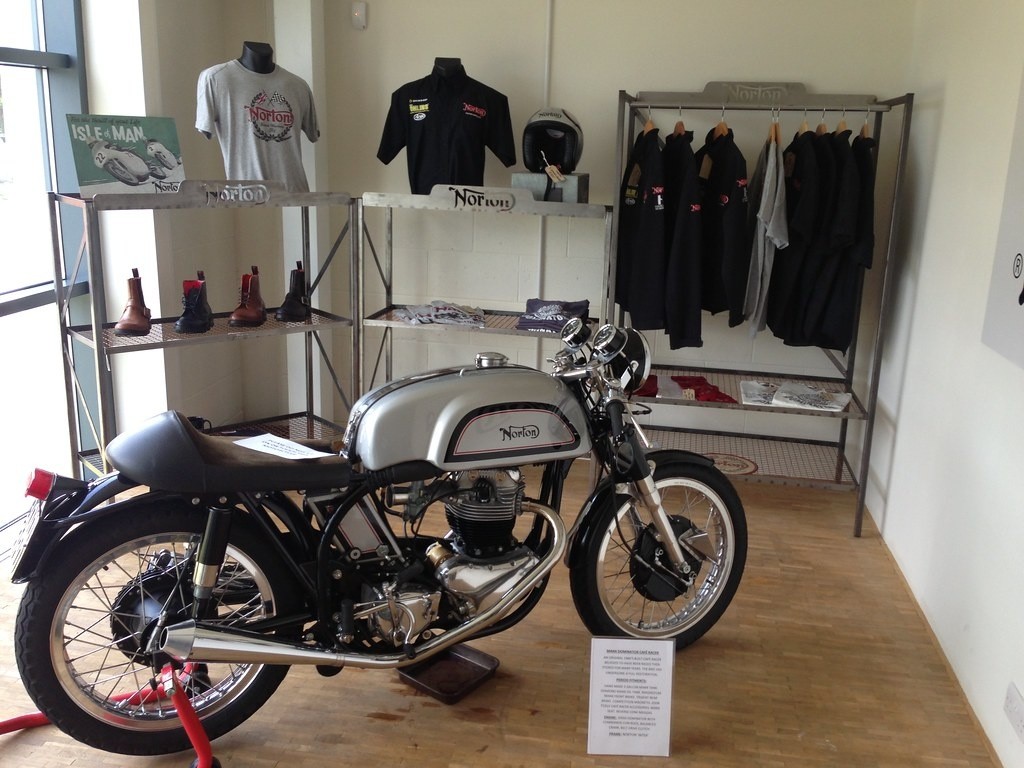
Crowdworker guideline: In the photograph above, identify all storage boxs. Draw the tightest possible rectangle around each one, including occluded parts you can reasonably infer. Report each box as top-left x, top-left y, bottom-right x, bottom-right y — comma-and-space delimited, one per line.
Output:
512, 172, 589, 204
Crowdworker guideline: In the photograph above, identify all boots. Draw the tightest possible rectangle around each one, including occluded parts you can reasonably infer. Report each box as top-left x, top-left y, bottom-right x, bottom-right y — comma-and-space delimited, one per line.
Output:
114, 268, 151, 336
174, 271, 214, 334
228, 266, 267, 327
276, 261, 311, 322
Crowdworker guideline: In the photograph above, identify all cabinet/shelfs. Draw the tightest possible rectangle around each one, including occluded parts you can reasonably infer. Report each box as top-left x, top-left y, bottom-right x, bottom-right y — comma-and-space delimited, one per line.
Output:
591, 79, 914, 538
48, 179, 359, 506
358, 193, 614, 461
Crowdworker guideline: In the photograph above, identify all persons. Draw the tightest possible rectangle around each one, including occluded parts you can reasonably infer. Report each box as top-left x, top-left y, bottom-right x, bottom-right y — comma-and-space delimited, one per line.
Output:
142, 136, 159, 150
85, 135, 123, 151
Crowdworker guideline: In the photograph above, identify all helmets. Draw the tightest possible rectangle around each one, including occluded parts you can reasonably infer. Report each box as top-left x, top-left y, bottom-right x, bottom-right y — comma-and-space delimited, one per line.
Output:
522, 107, 584, 175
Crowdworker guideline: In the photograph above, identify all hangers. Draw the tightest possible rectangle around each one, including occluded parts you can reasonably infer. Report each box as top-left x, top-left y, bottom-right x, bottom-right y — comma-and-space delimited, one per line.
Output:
637, 103, 877, 138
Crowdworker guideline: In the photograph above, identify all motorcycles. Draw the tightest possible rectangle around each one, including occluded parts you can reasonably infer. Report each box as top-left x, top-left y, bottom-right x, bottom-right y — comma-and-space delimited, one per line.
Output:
9, 301, 749, 757
